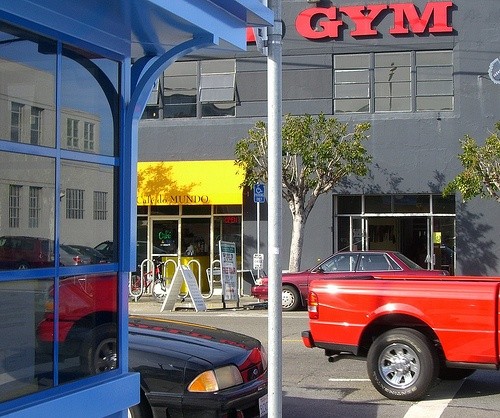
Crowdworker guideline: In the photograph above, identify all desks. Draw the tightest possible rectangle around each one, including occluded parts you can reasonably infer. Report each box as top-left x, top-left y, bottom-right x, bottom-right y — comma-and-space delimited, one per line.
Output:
161, 254, 210, 295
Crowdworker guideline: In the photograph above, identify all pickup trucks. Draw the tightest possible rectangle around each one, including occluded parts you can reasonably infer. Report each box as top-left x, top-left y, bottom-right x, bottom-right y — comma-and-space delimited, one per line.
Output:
36, 273, 118, 377
301, 275, 500, 402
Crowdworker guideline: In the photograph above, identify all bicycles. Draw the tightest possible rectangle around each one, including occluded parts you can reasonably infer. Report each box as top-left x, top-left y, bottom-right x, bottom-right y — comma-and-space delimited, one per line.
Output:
128, 257, 168, 303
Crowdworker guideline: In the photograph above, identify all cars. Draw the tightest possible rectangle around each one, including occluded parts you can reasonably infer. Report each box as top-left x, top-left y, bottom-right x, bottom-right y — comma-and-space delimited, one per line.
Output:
127, 314, 268, 418
251, 250, 451, 311
0, 234, 171, 279
